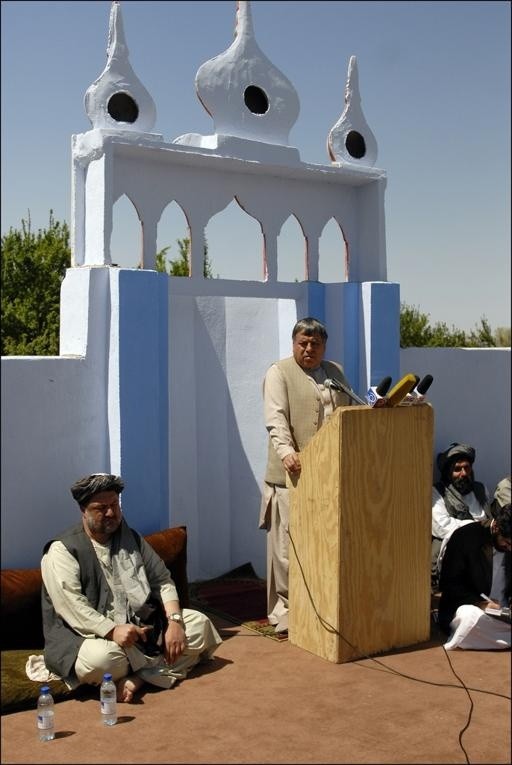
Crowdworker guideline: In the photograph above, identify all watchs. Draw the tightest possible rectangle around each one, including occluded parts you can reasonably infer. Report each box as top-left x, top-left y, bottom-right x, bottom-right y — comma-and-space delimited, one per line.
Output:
165, 613, 184, 624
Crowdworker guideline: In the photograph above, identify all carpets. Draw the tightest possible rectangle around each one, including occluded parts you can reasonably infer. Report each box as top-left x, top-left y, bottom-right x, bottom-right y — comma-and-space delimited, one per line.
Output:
188, 562, 290, 642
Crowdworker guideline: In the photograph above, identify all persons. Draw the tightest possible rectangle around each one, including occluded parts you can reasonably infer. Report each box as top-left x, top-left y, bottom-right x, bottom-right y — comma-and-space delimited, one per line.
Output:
40, 473, 223, 703
257, 310, 355, 639
431, 441, 512, 636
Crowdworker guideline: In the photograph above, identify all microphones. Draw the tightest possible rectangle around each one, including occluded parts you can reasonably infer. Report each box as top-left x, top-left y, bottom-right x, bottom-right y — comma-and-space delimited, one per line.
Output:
366, 375, 393, 407
324, 379, 344, 393
399, 374, 421, 407
412, 374, 434, 405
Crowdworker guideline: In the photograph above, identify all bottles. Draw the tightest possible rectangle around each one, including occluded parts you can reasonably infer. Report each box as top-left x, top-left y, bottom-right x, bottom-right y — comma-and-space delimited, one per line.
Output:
100, 673, 117, 725
38, 686, 56, 742
